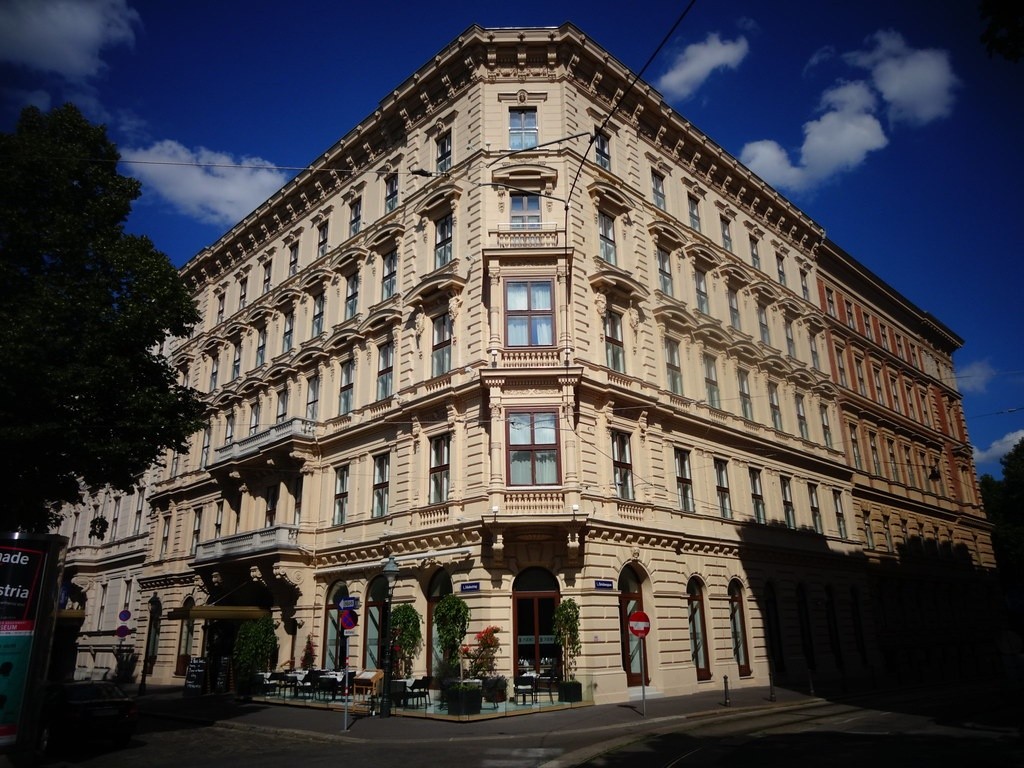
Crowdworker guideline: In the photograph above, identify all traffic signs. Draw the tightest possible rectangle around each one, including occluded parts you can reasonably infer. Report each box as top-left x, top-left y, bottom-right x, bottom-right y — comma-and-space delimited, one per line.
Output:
335, 595, 360, 614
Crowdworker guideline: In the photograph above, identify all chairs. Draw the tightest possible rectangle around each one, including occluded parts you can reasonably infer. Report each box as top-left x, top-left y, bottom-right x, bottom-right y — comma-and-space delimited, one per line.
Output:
249, 673, 555, 709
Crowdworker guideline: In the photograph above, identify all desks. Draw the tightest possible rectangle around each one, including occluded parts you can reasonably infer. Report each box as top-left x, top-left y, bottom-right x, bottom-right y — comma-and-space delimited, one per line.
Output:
258, 673, 277, 684
320, 674, 344, 693
463, 678, 483, 690
393, 679, 434, 705
285, 674, 313, 697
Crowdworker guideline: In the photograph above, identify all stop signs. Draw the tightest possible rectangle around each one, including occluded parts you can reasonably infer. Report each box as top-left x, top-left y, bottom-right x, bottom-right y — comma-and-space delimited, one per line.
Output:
628, 611, 651, 638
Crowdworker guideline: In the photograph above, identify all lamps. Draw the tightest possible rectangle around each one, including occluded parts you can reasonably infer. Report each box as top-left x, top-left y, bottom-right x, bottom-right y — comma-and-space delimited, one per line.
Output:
572, 504, 579, 514
492, 506, 499, 516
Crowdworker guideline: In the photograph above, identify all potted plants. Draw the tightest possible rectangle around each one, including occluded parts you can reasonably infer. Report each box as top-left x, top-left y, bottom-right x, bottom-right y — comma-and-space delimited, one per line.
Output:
551, 596, 583, 702
431, 592, 483, 715
232, 614, 280, 695
389, 601, 426, 708
